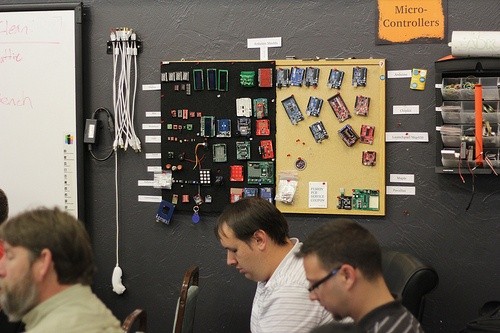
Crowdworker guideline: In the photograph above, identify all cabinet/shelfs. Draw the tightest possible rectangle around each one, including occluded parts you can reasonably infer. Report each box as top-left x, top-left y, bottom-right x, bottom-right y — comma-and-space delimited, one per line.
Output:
435, 56, 500, 174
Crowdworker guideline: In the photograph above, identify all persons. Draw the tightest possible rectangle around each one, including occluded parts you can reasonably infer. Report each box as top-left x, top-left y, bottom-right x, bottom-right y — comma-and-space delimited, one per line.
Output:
214, 195, 354, 333
0, 207, 129, 333
0, 187, 10, 269
294, 217, 425, 333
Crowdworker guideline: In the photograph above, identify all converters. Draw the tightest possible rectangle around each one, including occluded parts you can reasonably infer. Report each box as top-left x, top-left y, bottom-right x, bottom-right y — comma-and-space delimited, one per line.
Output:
84, 118, 98, 144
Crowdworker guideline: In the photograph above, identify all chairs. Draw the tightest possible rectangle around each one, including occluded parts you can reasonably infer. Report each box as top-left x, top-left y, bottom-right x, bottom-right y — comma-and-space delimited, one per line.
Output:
121, 267, 201, 333
381, 250, 438, 321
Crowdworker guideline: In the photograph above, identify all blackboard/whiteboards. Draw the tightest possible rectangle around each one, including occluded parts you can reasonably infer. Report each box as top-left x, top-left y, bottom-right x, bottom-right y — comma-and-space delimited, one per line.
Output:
0, 2, 83, 225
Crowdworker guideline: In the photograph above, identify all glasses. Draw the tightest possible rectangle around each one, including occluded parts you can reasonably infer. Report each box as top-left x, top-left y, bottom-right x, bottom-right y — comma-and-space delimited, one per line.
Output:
308, 265, 341, 293
